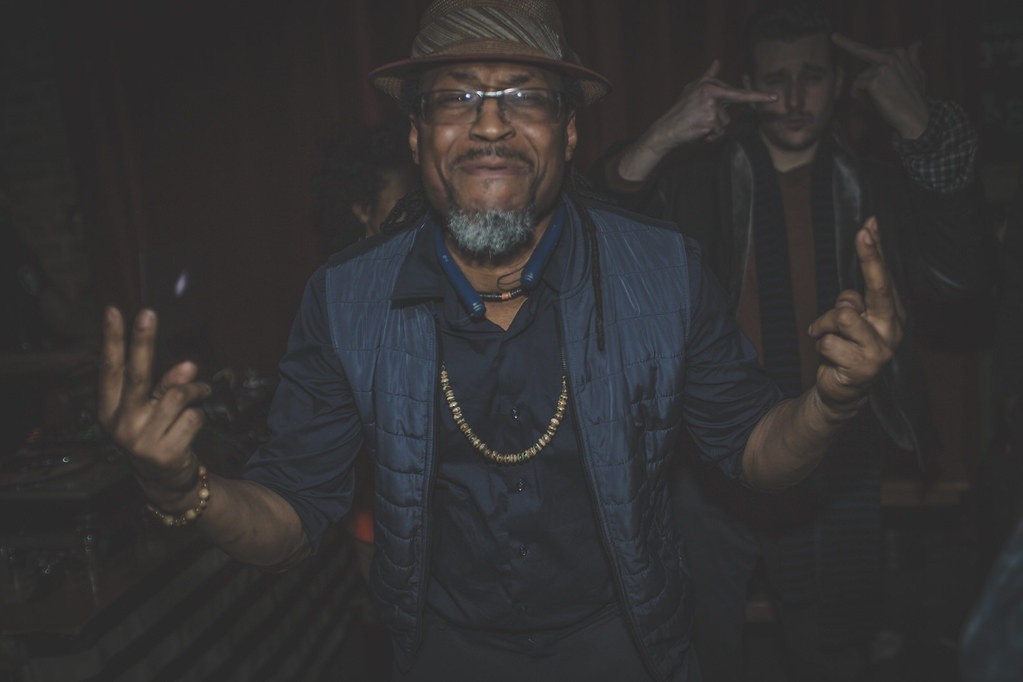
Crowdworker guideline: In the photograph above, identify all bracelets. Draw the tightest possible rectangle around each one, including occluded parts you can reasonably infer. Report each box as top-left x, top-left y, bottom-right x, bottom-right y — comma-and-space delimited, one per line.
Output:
146, 461, 210, 528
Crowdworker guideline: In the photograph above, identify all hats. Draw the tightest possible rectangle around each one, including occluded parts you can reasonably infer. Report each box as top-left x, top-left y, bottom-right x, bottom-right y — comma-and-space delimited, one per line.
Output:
370, 0, 613, 109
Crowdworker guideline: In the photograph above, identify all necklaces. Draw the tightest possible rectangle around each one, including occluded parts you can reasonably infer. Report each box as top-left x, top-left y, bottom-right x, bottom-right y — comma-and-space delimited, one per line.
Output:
478, 285, 527, 302
437, 356, 570, 465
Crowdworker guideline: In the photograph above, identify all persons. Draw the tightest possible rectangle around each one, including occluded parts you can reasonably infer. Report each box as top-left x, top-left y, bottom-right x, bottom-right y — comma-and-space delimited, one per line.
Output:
334, 119, 416, 584
96, 0, 907, 681
596, 0, 978, 681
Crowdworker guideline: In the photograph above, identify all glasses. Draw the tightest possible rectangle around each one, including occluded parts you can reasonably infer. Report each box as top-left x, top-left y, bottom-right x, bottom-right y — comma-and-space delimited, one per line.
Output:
410, 88, 571, 125
